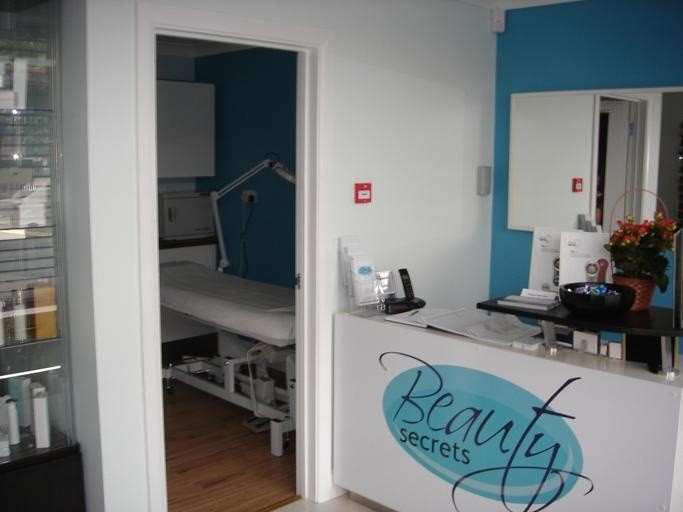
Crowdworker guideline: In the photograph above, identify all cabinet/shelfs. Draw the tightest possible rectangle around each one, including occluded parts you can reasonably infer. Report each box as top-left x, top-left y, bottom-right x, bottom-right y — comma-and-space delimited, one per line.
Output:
0, 3, 87, 511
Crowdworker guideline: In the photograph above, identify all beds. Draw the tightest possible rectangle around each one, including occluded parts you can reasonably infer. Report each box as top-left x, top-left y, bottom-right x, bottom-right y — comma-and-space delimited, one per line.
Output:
160, 260, 294, 457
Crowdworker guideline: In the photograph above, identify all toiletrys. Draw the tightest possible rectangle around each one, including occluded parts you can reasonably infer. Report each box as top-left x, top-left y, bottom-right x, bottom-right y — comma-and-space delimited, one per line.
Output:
0, 281, 56, 460
0, 55, 12, 91
11, 58, 28, 110
0, 89, 16, 110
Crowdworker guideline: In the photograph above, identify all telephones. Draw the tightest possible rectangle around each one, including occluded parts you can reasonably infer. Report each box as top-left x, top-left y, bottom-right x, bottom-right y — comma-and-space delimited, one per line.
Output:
384, 268, 426, 314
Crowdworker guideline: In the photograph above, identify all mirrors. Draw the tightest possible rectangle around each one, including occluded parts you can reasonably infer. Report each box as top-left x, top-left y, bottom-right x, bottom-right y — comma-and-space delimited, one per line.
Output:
593, 91, 682, 232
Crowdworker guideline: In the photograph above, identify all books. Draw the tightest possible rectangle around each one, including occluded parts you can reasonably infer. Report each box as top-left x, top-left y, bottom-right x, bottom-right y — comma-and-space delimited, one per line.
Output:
418, 306, 542, 347
558, 230, 616, 288
497, 295, 559, 312
527, 223, 584, 295
384, 307, 455, 329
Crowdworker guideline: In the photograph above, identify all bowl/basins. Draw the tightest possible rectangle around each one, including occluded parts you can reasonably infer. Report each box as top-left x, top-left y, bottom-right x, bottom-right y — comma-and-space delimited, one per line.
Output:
559, 280, 637, 316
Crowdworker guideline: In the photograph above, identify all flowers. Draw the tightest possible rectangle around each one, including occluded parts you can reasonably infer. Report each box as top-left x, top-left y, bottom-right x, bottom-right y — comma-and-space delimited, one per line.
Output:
602, 213, 675, 293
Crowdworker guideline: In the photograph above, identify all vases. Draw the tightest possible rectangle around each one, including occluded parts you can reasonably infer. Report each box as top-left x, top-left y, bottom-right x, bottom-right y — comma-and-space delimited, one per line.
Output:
610, 274, 653, 310
